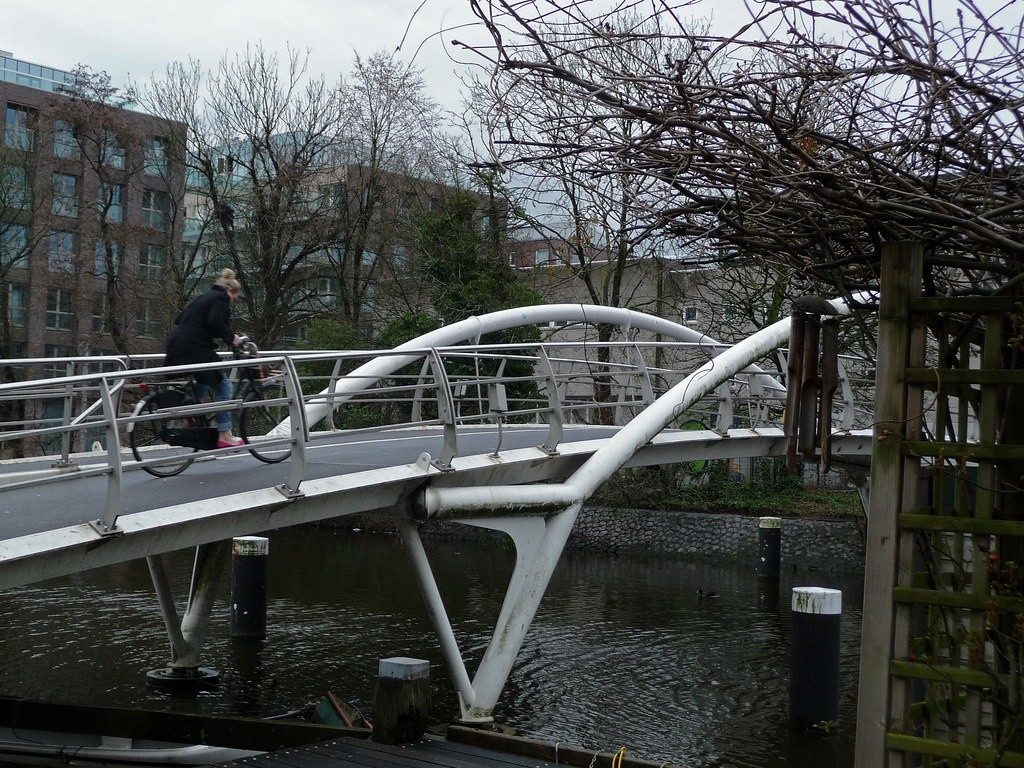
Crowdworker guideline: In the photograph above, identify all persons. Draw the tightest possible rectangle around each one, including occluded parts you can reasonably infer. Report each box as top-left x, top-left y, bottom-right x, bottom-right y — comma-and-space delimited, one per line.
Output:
166, 267, 244, 448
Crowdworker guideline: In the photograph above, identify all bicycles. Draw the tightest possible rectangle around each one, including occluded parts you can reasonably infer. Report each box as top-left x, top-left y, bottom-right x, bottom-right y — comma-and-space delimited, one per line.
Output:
126, 334, 308, 479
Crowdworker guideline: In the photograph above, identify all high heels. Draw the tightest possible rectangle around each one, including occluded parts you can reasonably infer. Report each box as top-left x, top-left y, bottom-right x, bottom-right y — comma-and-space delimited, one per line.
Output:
217, 440, 244, 456
